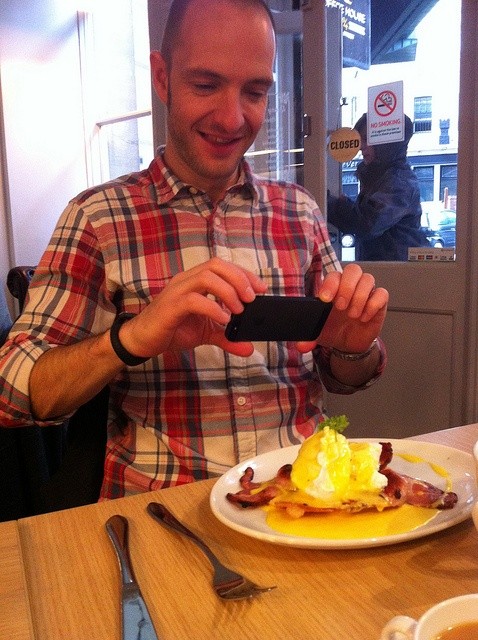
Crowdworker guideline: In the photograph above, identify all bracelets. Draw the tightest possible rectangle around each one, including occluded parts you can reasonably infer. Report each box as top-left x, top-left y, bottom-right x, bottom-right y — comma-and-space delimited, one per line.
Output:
110, 312, 147, 367
327, 340, 381, 363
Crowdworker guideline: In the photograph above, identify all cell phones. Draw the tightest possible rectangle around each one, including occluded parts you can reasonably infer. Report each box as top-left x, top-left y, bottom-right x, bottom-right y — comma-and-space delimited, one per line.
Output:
224, 295, 332, 343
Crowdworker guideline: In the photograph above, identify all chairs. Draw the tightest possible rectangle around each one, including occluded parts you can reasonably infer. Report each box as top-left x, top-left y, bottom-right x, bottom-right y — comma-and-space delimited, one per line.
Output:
6, 266, 107, 512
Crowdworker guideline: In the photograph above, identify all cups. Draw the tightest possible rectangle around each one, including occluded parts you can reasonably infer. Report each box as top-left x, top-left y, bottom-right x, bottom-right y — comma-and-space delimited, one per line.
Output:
381, 592, 477, 640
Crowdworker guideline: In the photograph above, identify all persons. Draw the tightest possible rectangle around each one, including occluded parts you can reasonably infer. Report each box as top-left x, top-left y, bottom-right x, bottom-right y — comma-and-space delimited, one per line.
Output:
0, 0, 390, 506
326, 111, 431, 263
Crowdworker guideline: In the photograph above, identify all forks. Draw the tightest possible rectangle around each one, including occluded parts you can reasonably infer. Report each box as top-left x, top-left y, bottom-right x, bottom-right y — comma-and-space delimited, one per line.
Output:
145, 501, 279, 602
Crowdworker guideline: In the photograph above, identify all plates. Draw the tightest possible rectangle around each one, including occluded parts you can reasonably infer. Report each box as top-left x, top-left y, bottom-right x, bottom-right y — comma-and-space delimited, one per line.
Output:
209, 437, 478, 553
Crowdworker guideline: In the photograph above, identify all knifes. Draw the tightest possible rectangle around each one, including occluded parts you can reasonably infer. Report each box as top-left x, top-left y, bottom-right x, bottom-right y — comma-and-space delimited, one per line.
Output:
104, 514, 161, 639
